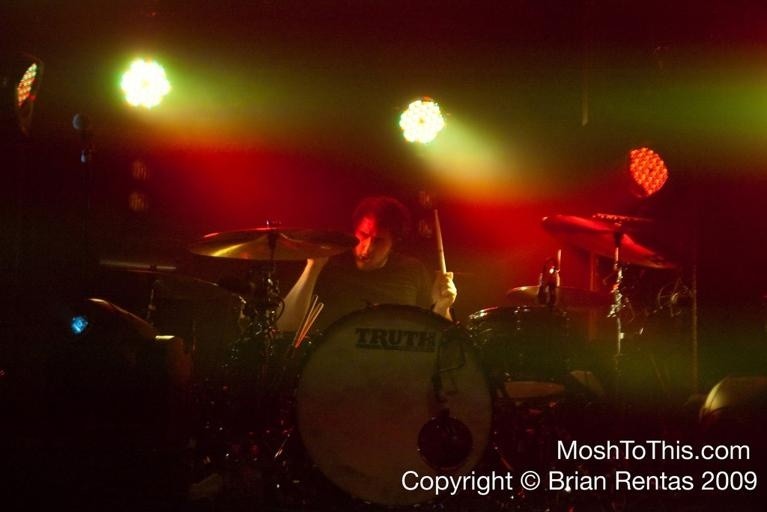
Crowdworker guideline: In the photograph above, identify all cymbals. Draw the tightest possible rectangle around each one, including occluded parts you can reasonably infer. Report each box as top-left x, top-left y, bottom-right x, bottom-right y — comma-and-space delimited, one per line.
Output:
505, 285, 606, 308
542, 212, 674, 270
90, 269, 228, 301
184, 227, 357, 262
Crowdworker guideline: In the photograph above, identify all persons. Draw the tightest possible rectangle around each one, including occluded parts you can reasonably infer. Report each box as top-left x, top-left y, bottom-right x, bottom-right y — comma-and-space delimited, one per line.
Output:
274, 195, 457, 338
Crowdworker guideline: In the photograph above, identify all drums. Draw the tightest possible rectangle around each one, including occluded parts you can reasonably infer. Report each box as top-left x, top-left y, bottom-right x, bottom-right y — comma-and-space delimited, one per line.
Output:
462, 304, 570, 399
278, 304, 492, 509
